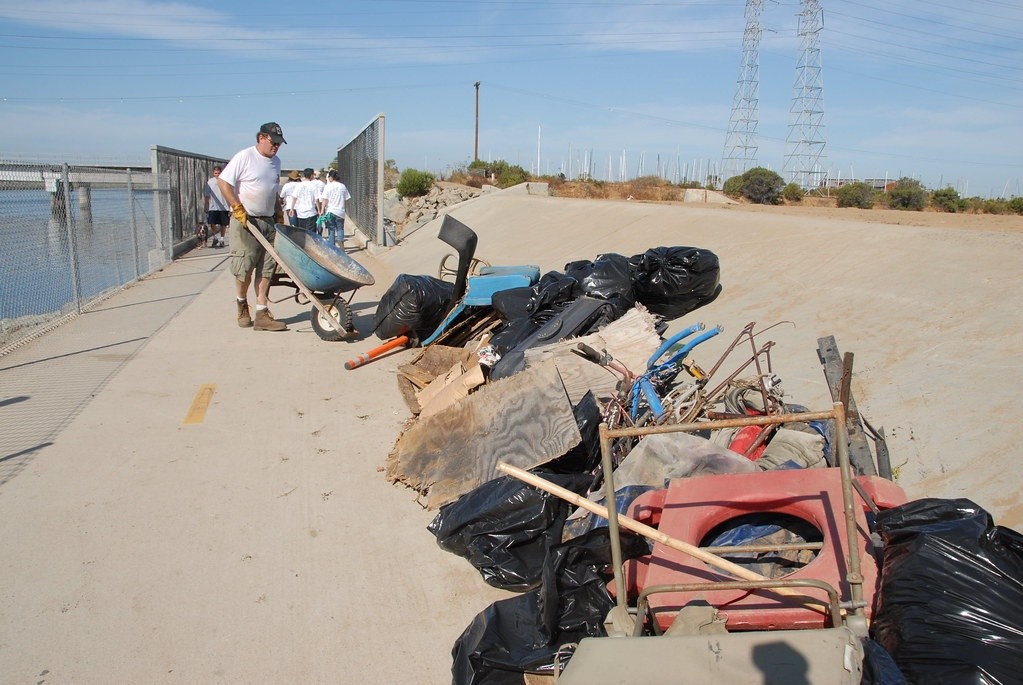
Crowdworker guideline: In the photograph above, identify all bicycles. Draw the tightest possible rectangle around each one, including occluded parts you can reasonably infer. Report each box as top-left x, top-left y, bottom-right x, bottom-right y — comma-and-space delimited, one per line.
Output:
561, 321, 783, 492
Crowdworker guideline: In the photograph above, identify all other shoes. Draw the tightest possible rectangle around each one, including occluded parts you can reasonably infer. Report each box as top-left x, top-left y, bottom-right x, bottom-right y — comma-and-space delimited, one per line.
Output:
220, 241, 224, 247
212, 238, 218, 247
339, 242, 346, 252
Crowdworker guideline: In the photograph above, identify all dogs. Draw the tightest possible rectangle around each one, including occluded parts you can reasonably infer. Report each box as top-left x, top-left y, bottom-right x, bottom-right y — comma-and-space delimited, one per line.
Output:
196, 223, 208, 249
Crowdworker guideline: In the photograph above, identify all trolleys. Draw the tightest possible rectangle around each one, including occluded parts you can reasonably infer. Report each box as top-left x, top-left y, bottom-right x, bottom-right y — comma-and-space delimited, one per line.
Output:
244, 219, 376, 343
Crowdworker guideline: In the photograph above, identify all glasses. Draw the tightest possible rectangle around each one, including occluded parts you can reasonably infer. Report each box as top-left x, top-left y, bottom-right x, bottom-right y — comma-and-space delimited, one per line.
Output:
262, 133, 281, 146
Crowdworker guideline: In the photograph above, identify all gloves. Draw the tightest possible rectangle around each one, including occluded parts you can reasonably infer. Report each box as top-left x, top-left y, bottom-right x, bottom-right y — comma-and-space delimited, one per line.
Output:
276, 210, 285, 224
232, 203, 249, 229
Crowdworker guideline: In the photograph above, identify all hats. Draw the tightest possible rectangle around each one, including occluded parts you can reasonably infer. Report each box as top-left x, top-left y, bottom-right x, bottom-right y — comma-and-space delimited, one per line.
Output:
261, 122, 287, 144
288, 171, 301, 180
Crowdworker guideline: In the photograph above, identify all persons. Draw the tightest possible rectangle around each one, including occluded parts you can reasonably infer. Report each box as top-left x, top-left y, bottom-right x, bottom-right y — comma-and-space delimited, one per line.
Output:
279, 168, 351, 253
217, 121, 287, 330
204, 166, 231, 248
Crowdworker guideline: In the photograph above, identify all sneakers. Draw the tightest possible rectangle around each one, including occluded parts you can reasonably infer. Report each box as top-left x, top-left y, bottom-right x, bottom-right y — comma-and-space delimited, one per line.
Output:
254, 308, 287, 331
237, 299, 252, 328
